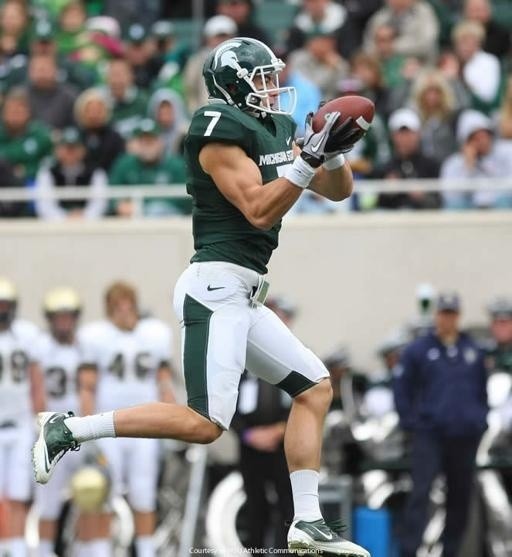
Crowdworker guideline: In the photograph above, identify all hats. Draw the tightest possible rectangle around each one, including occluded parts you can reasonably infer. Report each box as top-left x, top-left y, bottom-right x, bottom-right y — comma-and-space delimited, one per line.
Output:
456, 111, 492, 144
205, 16, 236, 38
437, 293, 459, 315
387, 108, 421, 134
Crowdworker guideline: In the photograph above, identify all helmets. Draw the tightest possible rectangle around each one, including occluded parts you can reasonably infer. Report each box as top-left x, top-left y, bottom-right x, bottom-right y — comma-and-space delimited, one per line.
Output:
0, 278, 16, 306
202, 37, 298, 116
39, 285, 80, 317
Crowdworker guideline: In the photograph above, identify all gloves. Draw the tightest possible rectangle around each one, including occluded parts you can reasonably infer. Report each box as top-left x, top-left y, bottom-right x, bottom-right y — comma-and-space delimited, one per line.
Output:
295, 98, 365, 169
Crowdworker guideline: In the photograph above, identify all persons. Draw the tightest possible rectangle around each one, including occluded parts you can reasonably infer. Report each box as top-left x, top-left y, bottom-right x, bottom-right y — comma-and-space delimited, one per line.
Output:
0, 0, 512, 557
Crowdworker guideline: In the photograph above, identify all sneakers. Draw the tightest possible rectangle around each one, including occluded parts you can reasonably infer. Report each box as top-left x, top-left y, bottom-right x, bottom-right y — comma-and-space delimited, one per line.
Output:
32, 411, 81, 484
287, 517, 371, 557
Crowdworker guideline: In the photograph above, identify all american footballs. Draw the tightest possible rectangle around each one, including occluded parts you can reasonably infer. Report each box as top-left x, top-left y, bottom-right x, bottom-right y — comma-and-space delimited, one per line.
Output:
308, 96, 374, 152
